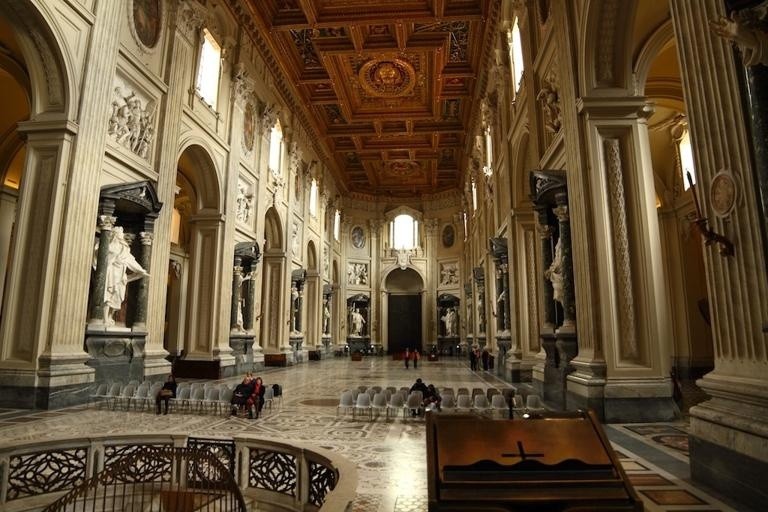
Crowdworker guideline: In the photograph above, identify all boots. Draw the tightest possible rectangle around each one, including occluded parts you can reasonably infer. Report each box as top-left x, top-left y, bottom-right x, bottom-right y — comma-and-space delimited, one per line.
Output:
253, 412, 259, 419
247, 410, 253, 419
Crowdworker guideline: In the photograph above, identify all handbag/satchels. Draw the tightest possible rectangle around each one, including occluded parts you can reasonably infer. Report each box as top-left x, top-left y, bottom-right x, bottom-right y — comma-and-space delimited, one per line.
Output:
160, 388, 171, 396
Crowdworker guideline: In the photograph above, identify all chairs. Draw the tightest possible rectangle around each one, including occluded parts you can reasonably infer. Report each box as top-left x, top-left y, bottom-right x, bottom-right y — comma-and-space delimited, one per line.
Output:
337, 385, 545, 422
89, 379, 286, 419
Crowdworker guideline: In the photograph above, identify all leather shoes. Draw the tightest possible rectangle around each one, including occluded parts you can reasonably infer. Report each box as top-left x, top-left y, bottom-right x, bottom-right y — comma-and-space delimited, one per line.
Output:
233, 407, 237, 413
230, 412, 237, 416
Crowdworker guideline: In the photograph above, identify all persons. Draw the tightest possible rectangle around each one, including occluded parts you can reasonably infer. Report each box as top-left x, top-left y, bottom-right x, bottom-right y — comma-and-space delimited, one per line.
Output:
409, 378, 442, 416
229, 371, 265, 419
546, 233, 564, 306
441, 308, 457, 336
102, 226, 147, 327
412, 348, 420, 368
469, 348, 489, 372
156, 375, 176, 415
404, 348, 410, 368
352, 307, 366, 336
